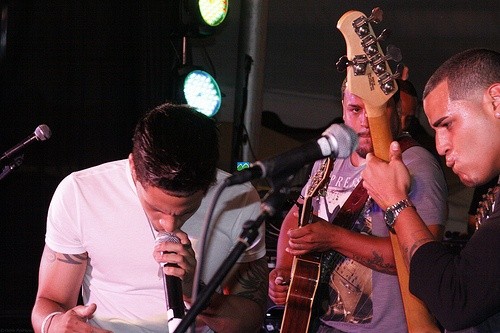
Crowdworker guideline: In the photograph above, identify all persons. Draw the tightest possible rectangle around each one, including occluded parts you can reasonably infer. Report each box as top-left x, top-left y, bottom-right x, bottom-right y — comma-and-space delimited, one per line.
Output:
268, 58, 448, 333
30, 104, 269, 333
359, 47, 500, 332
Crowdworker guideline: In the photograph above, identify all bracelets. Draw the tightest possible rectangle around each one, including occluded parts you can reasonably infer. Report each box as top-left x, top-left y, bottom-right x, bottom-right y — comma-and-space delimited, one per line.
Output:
41, 312, 63, 333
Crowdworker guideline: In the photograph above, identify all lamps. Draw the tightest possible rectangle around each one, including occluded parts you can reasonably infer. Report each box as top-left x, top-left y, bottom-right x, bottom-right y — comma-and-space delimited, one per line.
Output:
168, 0, 233, 123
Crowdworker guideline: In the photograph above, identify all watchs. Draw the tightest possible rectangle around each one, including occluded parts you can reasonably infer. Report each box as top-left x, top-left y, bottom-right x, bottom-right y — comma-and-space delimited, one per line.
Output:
384, 200, 416, 234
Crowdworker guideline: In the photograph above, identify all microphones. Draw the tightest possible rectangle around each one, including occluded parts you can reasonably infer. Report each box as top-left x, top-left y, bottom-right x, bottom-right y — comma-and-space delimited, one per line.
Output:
0, 124, 52, 167
224, 123, 358, 187
155, 230, 186, 333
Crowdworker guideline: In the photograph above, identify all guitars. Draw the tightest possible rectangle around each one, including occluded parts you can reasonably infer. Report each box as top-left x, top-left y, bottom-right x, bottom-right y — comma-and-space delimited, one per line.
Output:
336, 7, 446, 333
280, 158, 334, 333
